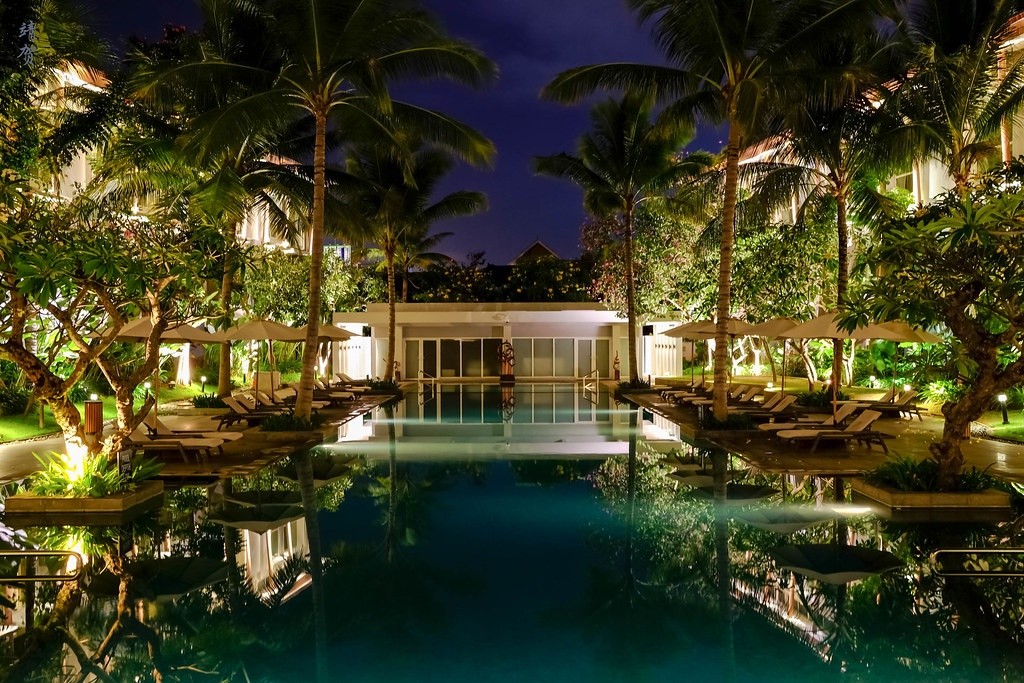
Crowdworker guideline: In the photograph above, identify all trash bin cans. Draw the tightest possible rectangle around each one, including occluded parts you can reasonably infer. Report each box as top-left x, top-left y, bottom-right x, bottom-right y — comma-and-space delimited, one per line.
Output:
84, 400, 103, 435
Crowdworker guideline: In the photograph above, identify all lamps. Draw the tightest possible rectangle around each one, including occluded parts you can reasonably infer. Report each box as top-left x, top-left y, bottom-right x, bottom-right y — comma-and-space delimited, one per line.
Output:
201, 376, 208, 382
870, 376, 875, 382
998, 393, 1007, 403
903, 384, 911, 392
144, 382, 151, 389
90, 393, 99, 400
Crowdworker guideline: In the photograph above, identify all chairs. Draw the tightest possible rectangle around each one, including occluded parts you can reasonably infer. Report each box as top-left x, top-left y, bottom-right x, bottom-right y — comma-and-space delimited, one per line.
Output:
110, 373, 372, 465
650, 376, 928, 456
